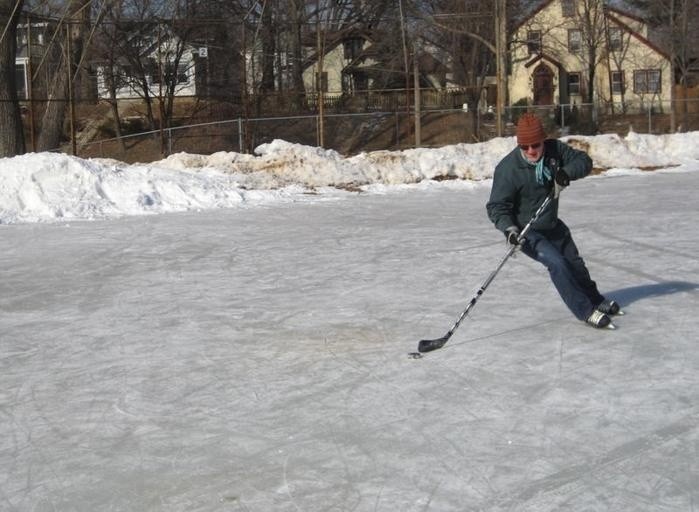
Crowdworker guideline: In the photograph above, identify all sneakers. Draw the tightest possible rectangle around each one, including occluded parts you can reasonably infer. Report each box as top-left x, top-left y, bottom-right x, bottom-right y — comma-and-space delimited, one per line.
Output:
598, 298, 619, 315
585, 308, 610, 328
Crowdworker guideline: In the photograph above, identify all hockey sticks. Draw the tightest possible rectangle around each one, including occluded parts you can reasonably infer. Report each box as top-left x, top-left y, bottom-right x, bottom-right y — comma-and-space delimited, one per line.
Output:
419, 185, 556, 353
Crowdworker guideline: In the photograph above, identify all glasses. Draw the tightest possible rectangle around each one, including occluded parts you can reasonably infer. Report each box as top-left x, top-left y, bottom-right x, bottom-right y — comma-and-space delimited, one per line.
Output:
518, 143, 540, 150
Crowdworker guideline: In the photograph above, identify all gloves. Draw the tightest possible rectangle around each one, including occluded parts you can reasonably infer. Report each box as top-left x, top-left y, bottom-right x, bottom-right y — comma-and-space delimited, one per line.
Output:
504, 225, 526, 258
555, 168, 569, 190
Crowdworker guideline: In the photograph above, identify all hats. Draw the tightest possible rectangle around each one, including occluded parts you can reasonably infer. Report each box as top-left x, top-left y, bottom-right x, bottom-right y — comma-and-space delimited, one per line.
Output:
517, 113, 544, 144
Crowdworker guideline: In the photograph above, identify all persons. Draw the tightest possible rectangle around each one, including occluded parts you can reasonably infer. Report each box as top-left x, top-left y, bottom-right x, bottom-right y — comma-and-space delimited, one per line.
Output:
483, 112, 621, 328
